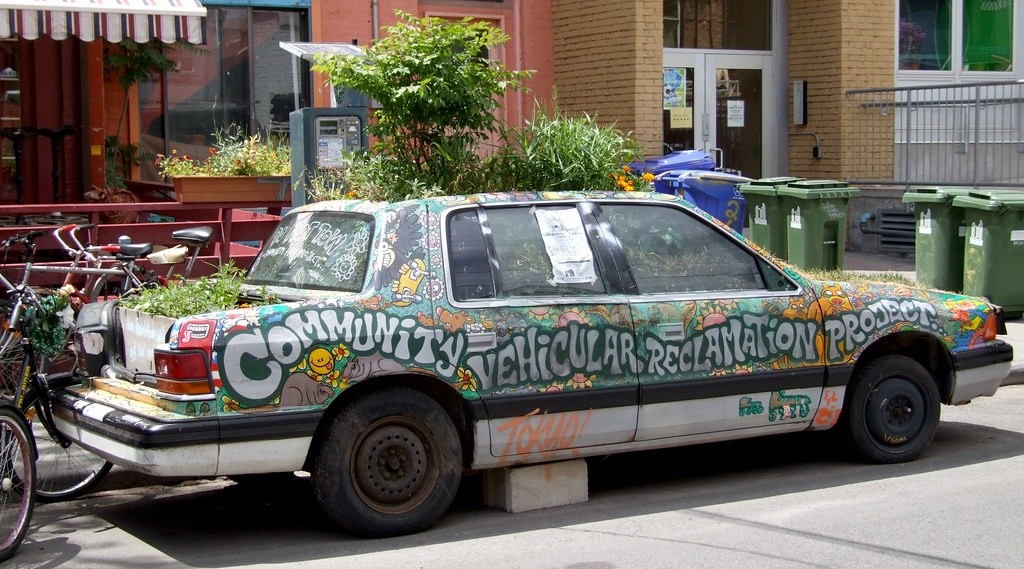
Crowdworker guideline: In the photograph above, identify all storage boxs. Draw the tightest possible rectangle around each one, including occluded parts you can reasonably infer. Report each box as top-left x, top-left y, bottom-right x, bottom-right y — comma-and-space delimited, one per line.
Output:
475, 459, 589, 512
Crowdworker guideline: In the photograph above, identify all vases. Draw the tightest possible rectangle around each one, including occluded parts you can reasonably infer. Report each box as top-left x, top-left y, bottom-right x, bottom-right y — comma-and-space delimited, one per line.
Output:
171, 173, 291, 203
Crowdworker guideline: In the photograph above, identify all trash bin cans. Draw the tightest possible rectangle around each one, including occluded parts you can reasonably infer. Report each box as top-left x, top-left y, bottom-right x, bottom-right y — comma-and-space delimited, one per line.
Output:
952, 188, 1024, 320
902, 186, 976, 294
655, 170, 754, 236
629, 150, 716, 176
777, 180, 861, 275
735, 177, 807, 265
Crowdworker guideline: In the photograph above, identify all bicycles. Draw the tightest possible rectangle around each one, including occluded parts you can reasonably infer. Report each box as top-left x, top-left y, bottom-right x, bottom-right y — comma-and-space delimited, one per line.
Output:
0, 224, 214, 560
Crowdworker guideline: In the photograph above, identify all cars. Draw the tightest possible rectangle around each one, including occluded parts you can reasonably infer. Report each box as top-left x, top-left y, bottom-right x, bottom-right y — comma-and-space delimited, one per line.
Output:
46, 191, 1013, 538
147, 25, 309, 147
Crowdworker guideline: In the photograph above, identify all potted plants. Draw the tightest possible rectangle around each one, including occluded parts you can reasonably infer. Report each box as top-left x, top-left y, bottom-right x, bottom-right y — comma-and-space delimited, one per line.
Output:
118, 256, 280, 376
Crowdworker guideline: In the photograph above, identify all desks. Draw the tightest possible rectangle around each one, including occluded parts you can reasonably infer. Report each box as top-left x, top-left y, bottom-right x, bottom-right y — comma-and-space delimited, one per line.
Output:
120, 179, 176, 202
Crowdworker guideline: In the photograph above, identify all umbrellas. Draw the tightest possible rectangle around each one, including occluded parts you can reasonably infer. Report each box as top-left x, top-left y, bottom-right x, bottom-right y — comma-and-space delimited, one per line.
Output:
148, 22, 305, 138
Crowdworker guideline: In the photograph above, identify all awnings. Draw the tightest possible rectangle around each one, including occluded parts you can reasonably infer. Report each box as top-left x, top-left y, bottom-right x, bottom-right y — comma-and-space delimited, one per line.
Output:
0, 0, 208, 45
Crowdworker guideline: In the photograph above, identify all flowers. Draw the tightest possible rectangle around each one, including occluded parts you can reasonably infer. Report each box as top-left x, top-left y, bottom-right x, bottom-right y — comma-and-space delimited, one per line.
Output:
153, 137, 282, 180
